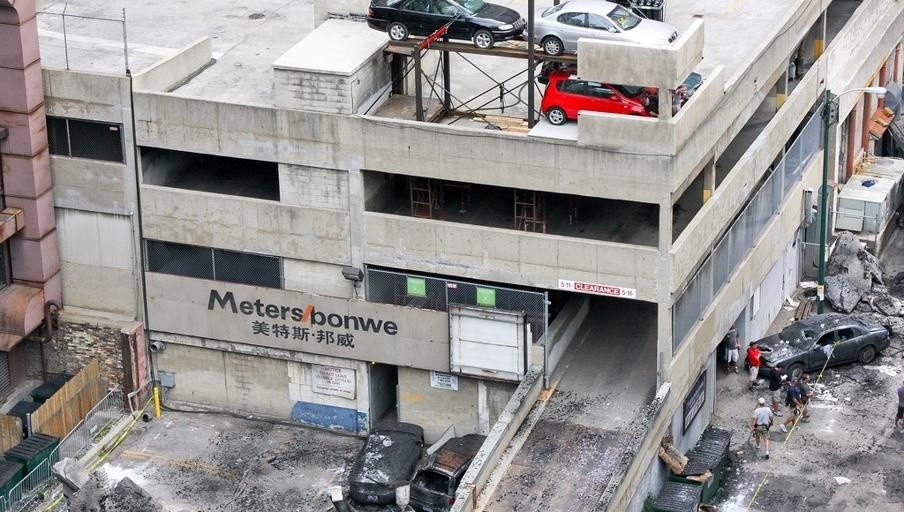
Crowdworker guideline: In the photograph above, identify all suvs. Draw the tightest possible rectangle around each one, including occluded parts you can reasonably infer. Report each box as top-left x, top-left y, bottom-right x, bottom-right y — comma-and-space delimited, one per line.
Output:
408, 434, 488, 511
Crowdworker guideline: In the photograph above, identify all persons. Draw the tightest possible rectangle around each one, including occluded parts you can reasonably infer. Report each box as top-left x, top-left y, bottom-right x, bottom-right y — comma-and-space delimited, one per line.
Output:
725, 325, 740, 374
769, 362, 784, 417
747, 341, 760, 391
895, 381, 904, 433
799, 373, 814, 421
751, 397, 773, 460
778, 376, 802, 432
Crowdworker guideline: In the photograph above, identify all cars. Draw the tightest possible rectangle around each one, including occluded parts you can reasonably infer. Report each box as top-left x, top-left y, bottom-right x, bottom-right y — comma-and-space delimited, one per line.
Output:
364, 0, 525, 50
535, 59, 704, 128
522, 0, 681, 55
745, 311, 890, 383
350, 418, 424, 504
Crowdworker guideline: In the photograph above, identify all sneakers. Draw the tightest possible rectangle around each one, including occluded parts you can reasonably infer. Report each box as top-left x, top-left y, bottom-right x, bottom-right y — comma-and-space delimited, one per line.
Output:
801, 414, 811, 423
735, 369, 740, 373
765, 450, 769, 459
795, 426, 801, 431
755, 443, 761, 450
779, 424, 788, 433
772, 408, 783, 417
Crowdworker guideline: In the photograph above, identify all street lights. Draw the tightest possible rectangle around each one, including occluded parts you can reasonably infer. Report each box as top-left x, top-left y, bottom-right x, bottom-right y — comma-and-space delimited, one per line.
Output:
817, 84, 888, 315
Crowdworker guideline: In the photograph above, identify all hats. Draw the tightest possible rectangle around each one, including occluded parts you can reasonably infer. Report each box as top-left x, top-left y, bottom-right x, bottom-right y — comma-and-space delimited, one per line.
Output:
758, 397, 765, 404
